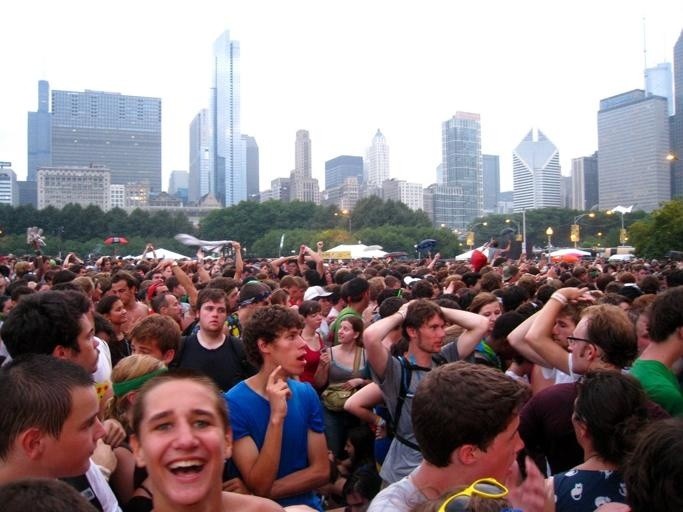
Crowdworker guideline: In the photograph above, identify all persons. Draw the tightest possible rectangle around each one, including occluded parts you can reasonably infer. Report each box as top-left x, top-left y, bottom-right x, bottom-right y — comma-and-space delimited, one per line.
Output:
2, 223, 682, 512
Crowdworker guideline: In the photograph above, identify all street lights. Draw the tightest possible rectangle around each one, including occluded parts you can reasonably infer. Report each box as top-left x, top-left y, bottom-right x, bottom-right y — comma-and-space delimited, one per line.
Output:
469, 222, 487, 251
546, 226, 554, 254
573, 213, 595, 248
606, 210, 625, 246
665, 154, 683, 161
505, 218, 521, 236
597, 232, 602, 248
440, 221, 463, 246
335, 209, 352, 232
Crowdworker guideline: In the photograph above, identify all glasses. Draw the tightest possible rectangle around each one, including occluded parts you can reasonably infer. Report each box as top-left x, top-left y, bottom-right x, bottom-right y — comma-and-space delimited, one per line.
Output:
568, 335, 598, 351
437, 478, 509, 512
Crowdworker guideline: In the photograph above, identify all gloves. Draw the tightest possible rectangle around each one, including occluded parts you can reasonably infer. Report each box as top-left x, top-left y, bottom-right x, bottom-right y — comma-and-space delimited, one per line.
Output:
373, 416, 387, 440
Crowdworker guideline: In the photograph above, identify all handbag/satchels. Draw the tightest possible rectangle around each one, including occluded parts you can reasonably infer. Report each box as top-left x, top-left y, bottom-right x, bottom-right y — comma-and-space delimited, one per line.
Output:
321, 383, 357, 411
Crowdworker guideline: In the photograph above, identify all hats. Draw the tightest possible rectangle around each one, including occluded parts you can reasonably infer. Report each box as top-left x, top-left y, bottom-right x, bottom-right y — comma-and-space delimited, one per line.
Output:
303, 286, 333, 301
404, 276, 422, 287
471, 250, 487, 271
502, 266, 521, 281
